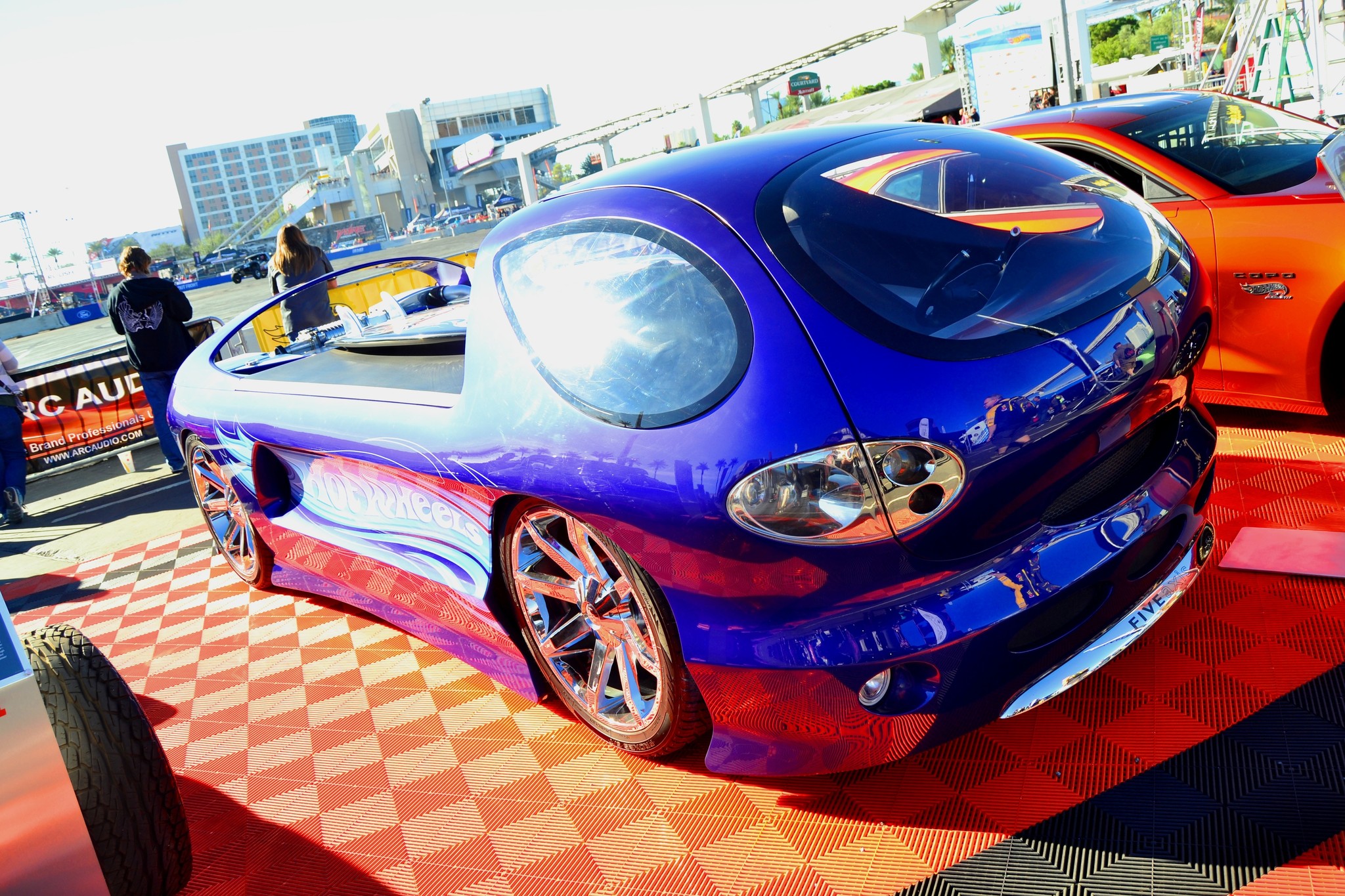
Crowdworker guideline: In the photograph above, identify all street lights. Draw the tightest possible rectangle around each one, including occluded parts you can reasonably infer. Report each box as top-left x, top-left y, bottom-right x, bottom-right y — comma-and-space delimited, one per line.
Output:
413, 172, 434, 223
536, 169, 545, 198
422, 98, 453, 216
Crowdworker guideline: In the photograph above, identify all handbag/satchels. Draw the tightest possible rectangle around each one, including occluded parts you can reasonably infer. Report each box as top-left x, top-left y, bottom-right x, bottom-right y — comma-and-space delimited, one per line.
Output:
14, 395, 27, 413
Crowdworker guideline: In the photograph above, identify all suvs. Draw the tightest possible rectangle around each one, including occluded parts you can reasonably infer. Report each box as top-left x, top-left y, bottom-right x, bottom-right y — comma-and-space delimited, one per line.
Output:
228, 252, 272, 284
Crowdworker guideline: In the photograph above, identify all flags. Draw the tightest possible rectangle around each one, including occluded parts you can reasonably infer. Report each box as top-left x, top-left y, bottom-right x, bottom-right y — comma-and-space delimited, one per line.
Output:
208, 221, 212, 233
1191, 2, 1202, 60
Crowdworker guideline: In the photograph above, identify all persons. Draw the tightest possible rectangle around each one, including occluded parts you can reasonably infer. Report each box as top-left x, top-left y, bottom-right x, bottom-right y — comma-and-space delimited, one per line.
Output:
108, 243, 197, 472
0, 307, 33, 319
910, 85, 1058, 132
387, 203, 528, 241
169, 273, 195, 283
288, 202, 295, 213
329, 233, 367, 250
0, 340, 30, 527
264, 222, 339, 341
306, 167, 395, 189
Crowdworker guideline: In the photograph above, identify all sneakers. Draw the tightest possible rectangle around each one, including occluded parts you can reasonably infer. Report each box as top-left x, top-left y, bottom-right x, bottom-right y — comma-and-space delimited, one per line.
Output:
2, 487, 25, 526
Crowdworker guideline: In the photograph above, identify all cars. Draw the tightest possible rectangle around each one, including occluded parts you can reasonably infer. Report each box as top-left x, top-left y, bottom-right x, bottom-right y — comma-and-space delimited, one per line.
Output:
427, 215, 476, 230
836, 91, 1345, 434
161, 124, 1226, 784
0, 583, 194, 895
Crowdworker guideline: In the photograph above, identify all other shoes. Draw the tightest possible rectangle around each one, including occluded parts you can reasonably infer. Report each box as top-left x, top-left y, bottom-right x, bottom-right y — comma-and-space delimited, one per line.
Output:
165, 457, 186, 472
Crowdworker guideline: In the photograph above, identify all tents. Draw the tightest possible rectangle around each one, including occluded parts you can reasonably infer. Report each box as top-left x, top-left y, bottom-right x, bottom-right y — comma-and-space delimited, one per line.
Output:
489, 192, 523, 215
